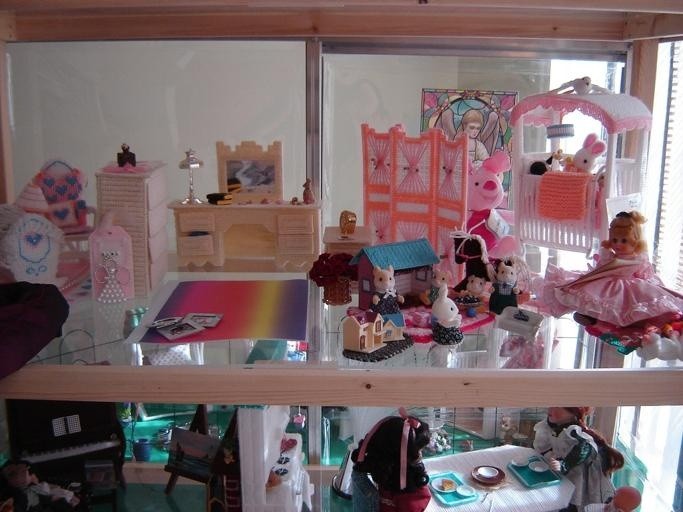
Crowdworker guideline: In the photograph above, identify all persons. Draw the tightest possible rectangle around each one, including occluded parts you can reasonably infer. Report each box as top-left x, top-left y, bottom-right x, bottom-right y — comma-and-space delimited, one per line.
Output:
532, 407, 625, 511
539, 210, 682, 354
452, 108, 504, 184
350, 405, 432, 512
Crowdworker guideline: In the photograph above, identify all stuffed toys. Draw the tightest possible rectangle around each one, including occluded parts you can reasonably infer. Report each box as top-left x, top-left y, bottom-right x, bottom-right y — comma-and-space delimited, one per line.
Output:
489, 261, 523, 316
528, 132, 607, 230
371, 264, 406, 314
636, 320, 683, 363
429, 281, 465, 352
452, 274, 486, 318
467, 150, 526, 267
418, 267, 453, 306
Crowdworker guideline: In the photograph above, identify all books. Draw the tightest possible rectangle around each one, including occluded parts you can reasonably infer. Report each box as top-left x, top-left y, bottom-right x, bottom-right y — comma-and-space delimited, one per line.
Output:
206, 192, 234, 201
208, 197, 234, 206
